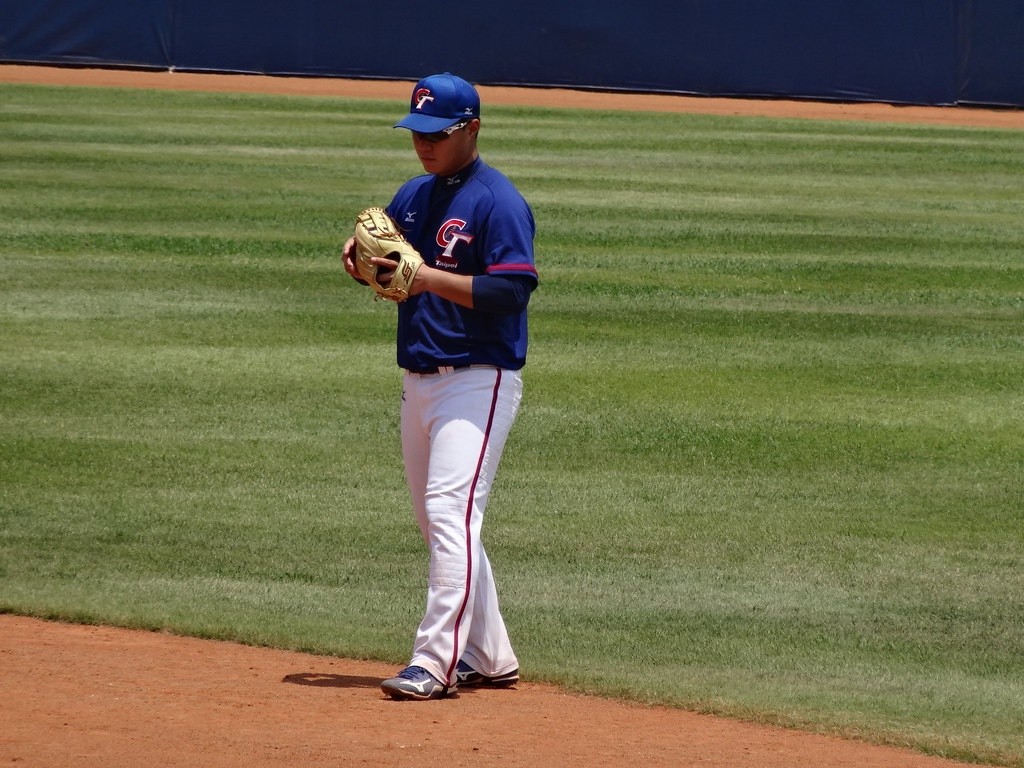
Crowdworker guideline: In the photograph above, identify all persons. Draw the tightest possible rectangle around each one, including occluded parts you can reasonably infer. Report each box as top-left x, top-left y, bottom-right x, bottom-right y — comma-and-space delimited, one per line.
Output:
341, 71, 538, 700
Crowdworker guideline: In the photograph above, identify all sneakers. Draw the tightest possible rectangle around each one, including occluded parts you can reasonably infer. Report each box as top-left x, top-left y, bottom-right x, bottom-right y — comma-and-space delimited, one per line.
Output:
455, 659, 520, 687
380, 665, 458, 700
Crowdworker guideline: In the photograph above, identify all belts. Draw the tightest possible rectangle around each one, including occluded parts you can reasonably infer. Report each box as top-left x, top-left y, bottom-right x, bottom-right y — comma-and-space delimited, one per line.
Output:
408, 365, 472, 373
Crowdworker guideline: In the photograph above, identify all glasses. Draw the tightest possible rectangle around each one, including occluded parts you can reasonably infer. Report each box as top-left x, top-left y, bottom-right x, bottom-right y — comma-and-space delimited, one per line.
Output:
411, 123, 467, 142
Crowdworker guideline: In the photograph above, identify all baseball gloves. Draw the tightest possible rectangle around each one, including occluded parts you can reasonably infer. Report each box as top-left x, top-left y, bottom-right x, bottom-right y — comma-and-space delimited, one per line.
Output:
352, 206, 426, 305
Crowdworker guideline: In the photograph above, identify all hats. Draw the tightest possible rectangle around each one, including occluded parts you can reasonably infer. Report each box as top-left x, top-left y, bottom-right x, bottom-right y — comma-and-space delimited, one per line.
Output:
393, 72, 480, 134
